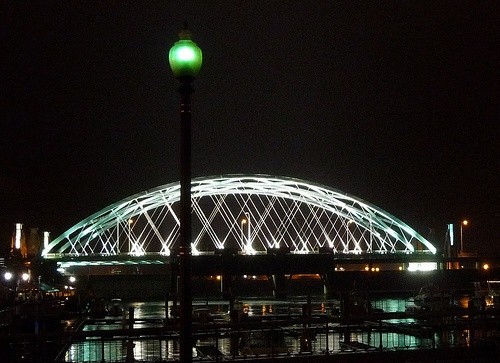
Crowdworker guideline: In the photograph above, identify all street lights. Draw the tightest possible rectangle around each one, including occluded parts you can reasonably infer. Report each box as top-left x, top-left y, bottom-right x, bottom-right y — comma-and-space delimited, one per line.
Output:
166, 26, 203, 359
460, 220, 467, 253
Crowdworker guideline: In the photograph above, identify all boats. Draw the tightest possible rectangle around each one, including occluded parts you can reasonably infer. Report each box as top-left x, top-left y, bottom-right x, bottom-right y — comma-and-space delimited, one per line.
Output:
108, 298, 125, 317
416, 284, 451, 306
89, 298, 111, 317
